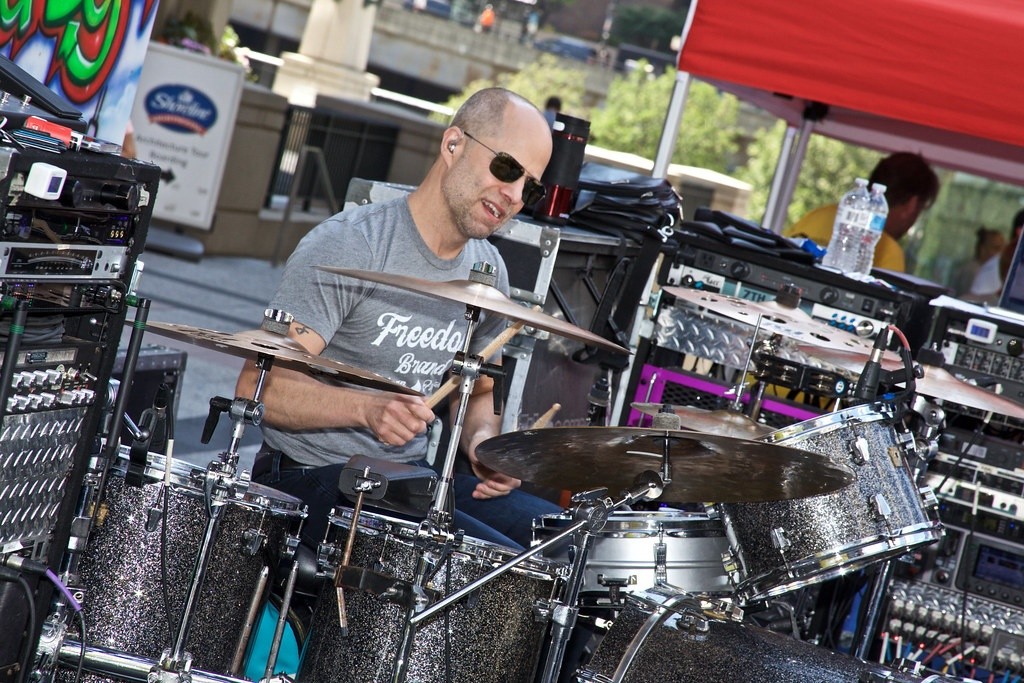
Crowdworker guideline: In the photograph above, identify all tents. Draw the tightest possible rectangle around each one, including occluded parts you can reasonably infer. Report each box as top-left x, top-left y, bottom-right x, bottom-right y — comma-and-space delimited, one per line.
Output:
652, 1, 1023, 232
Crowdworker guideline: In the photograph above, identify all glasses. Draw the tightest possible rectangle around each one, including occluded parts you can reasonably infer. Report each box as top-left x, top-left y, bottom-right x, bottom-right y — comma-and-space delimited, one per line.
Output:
464, 132, 547, 206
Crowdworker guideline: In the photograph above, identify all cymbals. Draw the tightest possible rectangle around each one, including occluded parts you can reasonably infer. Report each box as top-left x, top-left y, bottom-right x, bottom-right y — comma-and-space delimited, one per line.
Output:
310, 265, 636, 356
629, 400, 780, 441
127, 318, 426, 398
474, 427, 857, 503
792, 344, 1024, 421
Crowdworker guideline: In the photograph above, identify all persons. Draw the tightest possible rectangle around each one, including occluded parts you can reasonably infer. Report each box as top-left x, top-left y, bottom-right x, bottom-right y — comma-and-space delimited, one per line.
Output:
781, 153, 940, 272
972, 208, 1024, 302
947, 225, 1005, 299
232, 87, 568, 552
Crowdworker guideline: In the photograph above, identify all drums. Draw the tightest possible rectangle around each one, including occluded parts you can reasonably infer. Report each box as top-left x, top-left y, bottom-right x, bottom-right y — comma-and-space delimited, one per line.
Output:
537, 513, 730, 595
712, 402, 946, 601
573, 583, 961, 683
54, 444, 303, 682
295, 506, 567, 683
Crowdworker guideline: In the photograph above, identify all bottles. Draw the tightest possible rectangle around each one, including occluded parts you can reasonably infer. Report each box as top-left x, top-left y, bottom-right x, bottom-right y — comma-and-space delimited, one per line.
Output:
822, 178, 870, 273
532, 112, 592, 227
852, 183, 889, 274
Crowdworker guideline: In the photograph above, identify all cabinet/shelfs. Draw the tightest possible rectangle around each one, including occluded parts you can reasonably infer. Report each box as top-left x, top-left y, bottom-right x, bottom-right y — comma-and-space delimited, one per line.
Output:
343, 178, 677, 436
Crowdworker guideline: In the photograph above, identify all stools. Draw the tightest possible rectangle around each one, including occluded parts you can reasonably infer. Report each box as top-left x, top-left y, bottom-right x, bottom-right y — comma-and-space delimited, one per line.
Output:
268, 544, 320, 660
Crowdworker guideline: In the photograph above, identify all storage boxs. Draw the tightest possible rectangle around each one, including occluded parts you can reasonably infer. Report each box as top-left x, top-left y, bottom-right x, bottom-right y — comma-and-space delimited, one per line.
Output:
110, 340, 188, 455
